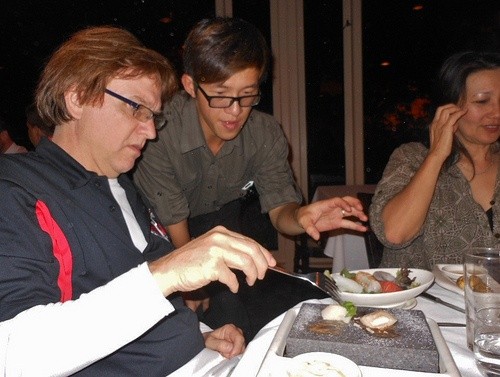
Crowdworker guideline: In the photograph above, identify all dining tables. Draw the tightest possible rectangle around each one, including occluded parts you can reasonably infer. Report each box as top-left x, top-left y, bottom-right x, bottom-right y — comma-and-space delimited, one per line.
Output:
228, 266, 500, 377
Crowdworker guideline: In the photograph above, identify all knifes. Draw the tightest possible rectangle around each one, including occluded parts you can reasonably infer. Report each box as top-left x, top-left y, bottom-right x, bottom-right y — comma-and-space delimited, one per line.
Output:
420, 292, 465, 314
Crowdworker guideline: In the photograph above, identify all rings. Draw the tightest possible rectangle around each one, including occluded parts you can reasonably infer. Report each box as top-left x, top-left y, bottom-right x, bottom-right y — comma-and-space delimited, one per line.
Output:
342, 210, 346, 218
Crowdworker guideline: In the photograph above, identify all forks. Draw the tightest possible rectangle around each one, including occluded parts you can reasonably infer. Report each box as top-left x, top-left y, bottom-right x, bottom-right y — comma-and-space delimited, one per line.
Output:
267, 265, 343, 306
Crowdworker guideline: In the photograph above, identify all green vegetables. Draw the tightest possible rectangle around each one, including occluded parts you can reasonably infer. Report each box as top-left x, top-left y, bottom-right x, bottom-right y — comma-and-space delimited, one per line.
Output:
340, 300, 357, 317
340, 268, 356, 279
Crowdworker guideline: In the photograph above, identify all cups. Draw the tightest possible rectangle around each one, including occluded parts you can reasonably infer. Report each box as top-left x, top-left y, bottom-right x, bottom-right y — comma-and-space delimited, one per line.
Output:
284, 352, 363, 377
463, 247, 500, 377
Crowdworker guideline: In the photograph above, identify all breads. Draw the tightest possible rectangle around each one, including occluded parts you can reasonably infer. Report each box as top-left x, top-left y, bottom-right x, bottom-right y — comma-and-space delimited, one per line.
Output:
455, 275, 482, 290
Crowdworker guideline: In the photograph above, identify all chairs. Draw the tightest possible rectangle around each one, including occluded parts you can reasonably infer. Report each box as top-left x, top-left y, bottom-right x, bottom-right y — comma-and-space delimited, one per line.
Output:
293, 183, 379, 276
355, 191, 386, 269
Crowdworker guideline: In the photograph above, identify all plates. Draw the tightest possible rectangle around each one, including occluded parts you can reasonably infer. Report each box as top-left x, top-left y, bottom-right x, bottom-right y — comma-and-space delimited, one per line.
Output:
441, 265, 488, 280
331, 267, 435, 308
436, 263, 500, 297
256, 307, 461, 377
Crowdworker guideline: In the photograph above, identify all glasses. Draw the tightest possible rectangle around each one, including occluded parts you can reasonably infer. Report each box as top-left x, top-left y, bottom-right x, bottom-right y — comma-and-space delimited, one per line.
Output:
196, 81, 262, 109
104, 88, 167, 129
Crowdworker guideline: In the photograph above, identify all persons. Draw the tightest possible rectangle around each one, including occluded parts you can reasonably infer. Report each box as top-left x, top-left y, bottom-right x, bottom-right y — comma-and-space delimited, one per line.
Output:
0, 26, 278, 377
131, 17, 368, 344
0, 120, 28, 154
368, 51, 500, 270
26, 104, 54, 146
